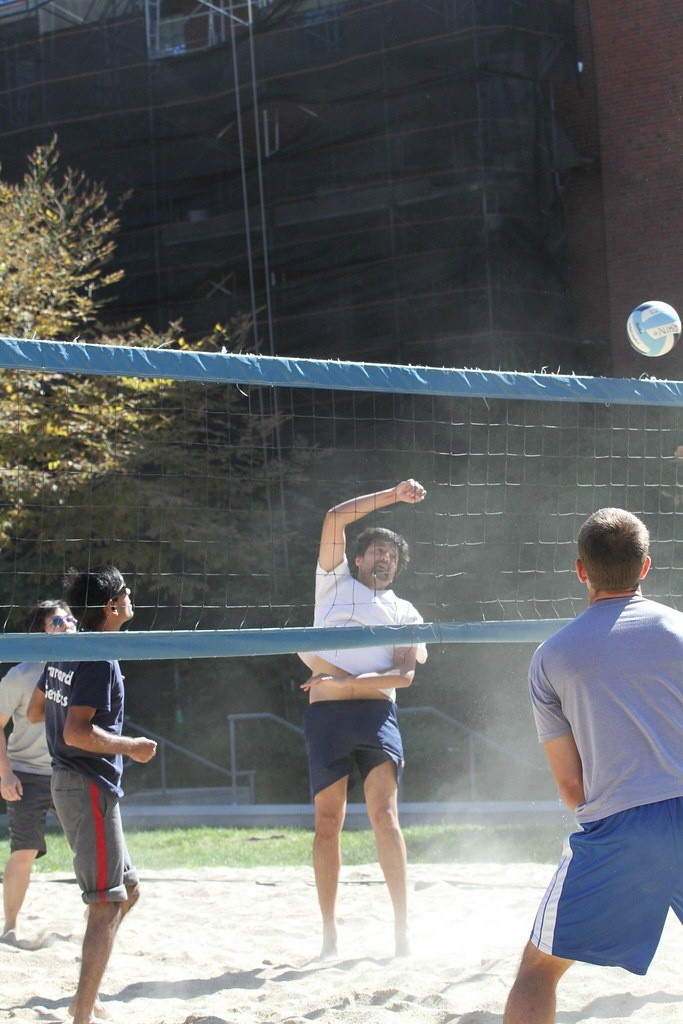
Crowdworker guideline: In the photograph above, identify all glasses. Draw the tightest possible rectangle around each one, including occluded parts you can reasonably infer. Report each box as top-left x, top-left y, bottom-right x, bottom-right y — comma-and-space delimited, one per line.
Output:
105, 582, 128, 607
43, 614, 78, 630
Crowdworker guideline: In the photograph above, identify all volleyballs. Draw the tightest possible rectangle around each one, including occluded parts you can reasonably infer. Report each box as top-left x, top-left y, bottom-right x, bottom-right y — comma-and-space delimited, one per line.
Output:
625, 299, 683, 357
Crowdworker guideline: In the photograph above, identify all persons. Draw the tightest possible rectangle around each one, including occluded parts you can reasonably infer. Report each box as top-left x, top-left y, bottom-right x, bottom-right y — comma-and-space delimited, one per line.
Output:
26, 567, 158, 1023
0, 601, 77, 946
298, 480, 429, 960
502, 508, 683, 1023
